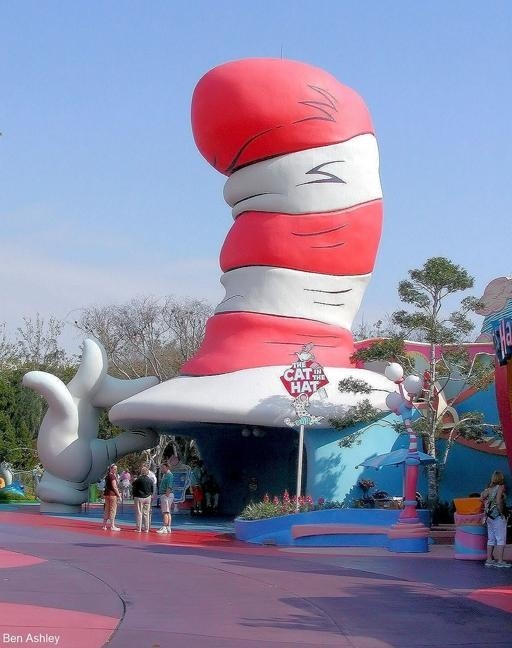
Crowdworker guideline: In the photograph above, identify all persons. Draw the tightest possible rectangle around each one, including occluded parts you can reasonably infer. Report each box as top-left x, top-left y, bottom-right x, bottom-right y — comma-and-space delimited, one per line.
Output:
189, 459, 205, 514
203, 476, 220, 510
155, 461, 175, 534
481, 470, 511, 567
100, 463, 158, 498
99, 463, 122, 531
483, 479, 508, 563
131, 467, 155, 534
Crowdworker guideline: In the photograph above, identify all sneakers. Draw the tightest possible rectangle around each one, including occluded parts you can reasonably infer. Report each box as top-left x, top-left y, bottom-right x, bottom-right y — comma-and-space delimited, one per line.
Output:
484, 560, 511, 568
100, 526, 172, 534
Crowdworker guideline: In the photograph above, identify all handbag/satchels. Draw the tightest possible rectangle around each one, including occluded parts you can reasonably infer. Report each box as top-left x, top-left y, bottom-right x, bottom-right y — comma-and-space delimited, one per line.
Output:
486, 495, 507, 520
